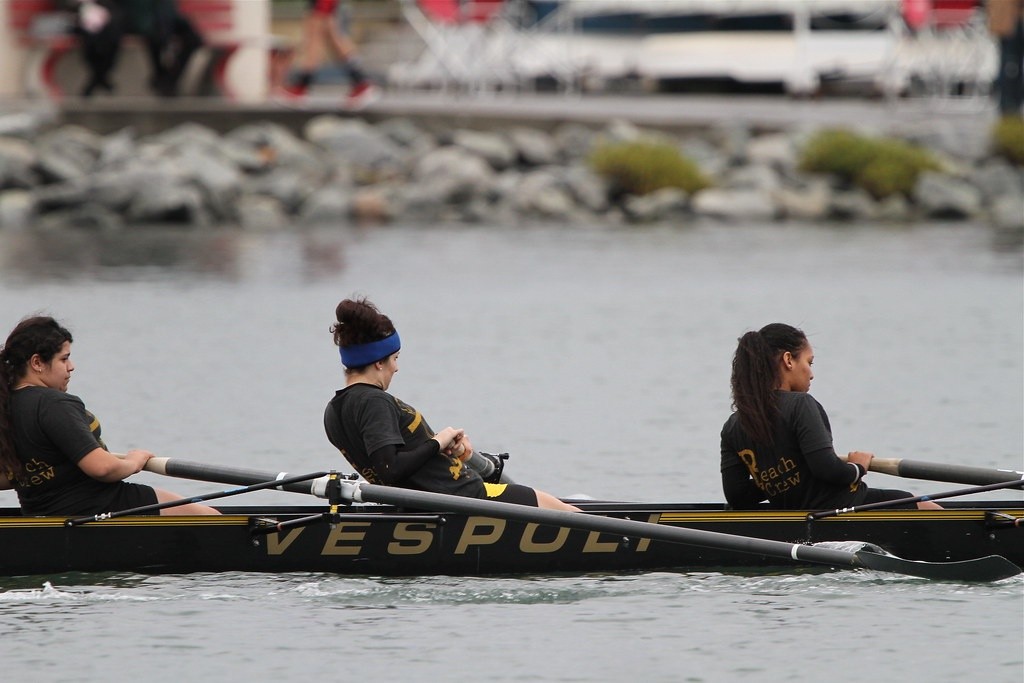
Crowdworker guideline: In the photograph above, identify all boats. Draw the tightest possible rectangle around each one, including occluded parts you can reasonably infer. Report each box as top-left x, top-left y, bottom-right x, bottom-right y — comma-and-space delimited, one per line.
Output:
0, 499, 1024, 575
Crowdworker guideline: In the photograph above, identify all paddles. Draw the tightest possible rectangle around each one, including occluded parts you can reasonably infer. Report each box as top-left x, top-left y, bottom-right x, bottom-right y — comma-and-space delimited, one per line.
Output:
840, 456, 1023, 489
450, 442, 517, 484
112, 453, 1022, 584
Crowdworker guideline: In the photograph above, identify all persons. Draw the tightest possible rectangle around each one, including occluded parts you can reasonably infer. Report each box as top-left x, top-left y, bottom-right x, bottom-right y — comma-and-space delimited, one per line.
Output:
0, 316, 222, 515
324, 300, 583, 512
282, 0, 371, 98
76, 0, 198, 97
720, 322, 943, 511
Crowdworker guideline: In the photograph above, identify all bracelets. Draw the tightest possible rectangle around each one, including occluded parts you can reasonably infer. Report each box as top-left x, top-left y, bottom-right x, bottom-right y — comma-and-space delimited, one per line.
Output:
848, 463, 860, 483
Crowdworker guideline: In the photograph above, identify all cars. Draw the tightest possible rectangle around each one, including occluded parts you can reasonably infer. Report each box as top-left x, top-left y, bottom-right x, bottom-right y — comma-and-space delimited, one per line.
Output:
500, 1, 1001, 99
